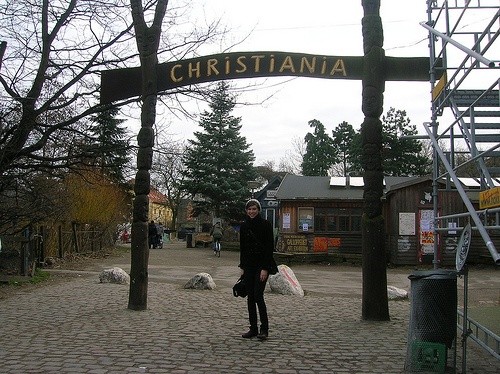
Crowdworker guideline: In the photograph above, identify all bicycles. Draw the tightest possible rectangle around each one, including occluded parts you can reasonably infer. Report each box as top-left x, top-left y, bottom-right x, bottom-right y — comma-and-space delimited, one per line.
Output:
156, 236, 163, 249
215, 238, 221, 257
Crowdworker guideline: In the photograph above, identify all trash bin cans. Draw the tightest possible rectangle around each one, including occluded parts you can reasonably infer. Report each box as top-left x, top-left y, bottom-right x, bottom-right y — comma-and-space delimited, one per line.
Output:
410, 269, 457, 345
185, 233, 196, 247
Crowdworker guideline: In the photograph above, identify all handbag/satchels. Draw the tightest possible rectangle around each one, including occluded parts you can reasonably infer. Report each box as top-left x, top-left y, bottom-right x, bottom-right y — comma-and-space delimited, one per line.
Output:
232, 274, 247, 298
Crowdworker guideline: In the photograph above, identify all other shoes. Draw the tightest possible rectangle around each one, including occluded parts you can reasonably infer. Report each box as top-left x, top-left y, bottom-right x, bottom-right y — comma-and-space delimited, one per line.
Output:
257, 324, 268, 338
242, 326, 258, 338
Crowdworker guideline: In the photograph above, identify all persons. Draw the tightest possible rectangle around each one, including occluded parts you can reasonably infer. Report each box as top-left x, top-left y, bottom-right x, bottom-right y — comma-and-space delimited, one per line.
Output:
156, 222, 164, 244
210, 221, 224, 254
121, 230, 128, 242
238, 199, 279, 339
148, 220, 159, 249
